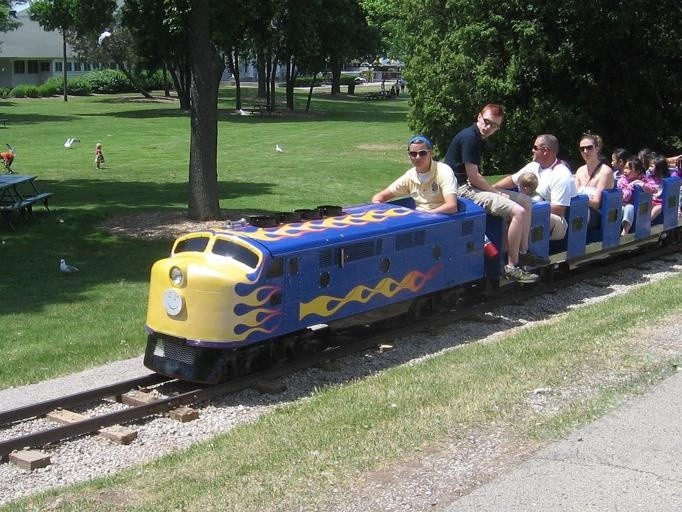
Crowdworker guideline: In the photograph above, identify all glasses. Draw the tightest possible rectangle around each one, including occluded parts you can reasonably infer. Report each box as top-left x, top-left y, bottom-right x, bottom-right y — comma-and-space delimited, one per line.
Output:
408, 149, 431, 157
482, 115, 501, 130
532, 144, 551, 151
579, 143, 596, 152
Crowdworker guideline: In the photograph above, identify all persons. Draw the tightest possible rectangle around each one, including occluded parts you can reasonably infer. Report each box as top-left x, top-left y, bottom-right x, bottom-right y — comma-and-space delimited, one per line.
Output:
517, 172, 544, 204
370, 134, 461, 217
574, 129, 681, 241
0, 150, 17, 174
491, 133, 575, 242
95, 142, 106, 170
380, 79, 407, 98
442, 102, 552, 285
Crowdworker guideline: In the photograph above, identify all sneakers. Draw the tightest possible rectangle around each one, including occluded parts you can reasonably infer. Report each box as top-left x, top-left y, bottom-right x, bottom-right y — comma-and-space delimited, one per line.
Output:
504, 264, 539, 284
518, 250, 551, 267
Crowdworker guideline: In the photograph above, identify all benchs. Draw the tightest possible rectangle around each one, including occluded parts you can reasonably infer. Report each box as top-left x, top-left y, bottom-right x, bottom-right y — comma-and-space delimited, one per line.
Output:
0, 193, 52, 231
250, 110, 282, 118
364, 94, 392, 100
0, 119, 8, 128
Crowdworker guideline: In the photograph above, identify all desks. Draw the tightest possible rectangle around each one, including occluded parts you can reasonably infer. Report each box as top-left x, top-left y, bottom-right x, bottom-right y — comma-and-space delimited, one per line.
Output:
0, 174, 51, 231
250, 104, 280, 117
385, 91, 390, 94
0, 113, 5, 114
367, 92, 380, 100
